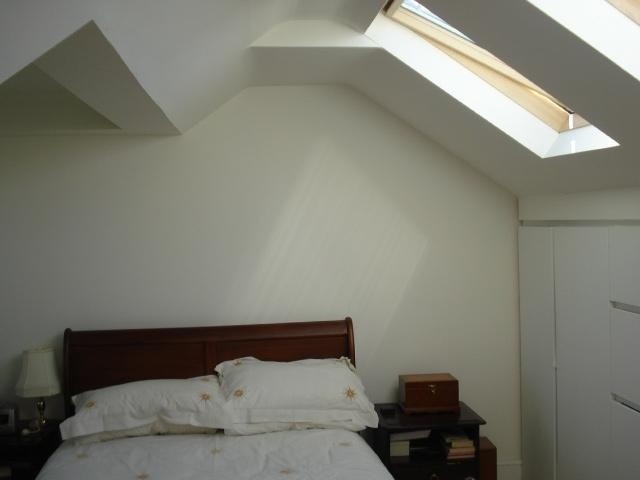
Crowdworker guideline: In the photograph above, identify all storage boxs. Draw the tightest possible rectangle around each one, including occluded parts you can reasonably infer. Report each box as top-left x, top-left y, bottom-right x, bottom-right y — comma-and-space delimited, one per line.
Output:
397, 372, 460, 415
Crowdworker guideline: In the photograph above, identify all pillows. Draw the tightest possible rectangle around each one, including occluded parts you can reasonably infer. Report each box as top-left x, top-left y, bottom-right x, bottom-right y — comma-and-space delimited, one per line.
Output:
57, 374, 222, 445
214, 355, 379, 429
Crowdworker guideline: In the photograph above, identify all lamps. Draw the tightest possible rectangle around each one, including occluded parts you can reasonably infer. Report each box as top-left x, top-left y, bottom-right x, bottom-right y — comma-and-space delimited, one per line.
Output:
14, 347, 62, 426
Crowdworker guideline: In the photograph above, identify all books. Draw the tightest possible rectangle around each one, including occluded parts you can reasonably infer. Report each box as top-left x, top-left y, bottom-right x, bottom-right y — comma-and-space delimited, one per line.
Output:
390, 429, 476, 460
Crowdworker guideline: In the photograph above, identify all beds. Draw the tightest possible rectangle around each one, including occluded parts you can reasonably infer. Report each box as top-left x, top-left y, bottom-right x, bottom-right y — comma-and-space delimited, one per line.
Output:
33, 316, 395, 480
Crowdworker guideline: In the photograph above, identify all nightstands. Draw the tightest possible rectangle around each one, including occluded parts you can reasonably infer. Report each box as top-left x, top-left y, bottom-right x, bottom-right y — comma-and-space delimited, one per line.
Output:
366, 402, 487, 480
0, 421, 61, 480
